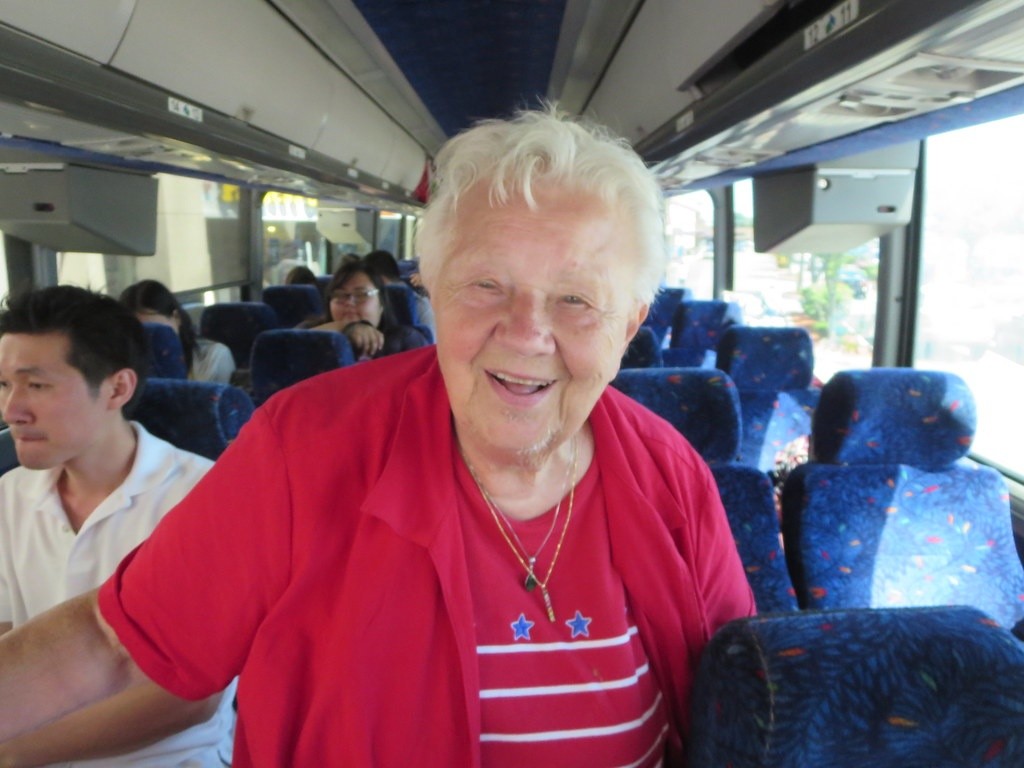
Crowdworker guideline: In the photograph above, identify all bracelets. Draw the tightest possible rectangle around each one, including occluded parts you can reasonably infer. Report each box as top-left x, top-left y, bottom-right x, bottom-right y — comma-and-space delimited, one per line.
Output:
342, 320, 372, 337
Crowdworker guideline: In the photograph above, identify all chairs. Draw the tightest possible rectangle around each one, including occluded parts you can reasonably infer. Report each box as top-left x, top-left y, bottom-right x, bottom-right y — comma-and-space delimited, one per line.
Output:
0, 259, 1024, 768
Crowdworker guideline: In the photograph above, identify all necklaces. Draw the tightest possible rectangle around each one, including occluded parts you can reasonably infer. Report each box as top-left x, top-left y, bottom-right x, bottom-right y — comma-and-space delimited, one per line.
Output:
461, 454, 579, 622
464, 442, 574, 589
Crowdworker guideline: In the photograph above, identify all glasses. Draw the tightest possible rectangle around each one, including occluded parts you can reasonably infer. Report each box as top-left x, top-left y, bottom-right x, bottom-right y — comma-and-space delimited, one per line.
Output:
329, 290, 380, 304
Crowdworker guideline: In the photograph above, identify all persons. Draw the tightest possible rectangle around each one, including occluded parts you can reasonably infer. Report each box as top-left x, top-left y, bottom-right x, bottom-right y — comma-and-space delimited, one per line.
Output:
366, 249, 437, 344
0, 108, 755, 768
290, 255, 429, 363
286, 266, 320, 285
0, 286, 237, 768
118, 279, 236, 386
